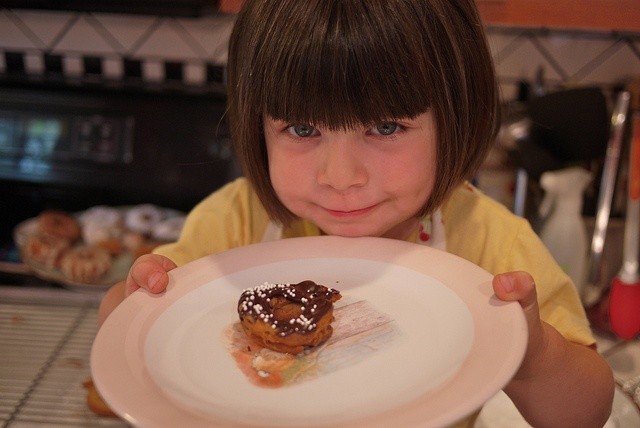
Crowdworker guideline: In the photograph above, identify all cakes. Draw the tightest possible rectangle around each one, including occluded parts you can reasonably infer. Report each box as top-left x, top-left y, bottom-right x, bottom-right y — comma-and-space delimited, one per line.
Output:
230, 281, 394, 386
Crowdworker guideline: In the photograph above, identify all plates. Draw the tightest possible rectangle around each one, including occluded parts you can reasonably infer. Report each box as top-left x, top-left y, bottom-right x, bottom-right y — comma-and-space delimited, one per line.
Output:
17, 214, 133, 291
91, 237, 528, 428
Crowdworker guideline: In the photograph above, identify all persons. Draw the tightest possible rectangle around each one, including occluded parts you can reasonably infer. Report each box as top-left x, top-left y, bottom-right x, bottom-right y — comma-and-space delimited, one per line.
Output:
96, 0, 614, 428
